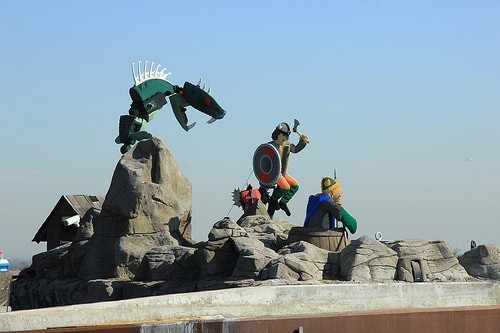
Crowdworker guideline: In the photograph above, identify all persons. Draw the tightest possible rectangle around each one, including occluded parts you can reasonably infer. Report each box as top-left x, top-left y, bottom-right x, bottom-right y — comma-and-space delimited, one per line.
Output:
302, 176, 357, 234
260, 122, 309, 217
